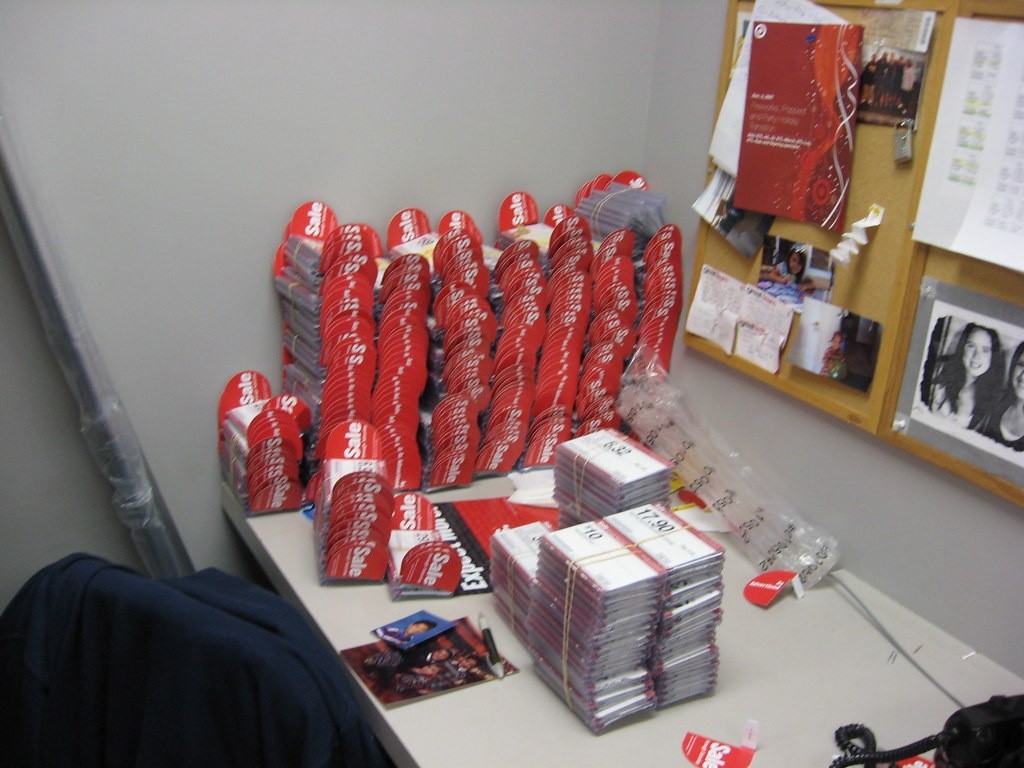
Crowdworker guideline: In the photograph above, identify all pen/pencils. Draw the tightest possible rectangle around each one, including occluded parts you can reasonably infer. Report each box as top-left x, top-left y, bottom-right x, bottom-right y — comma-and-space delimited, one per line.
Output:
474, 611, 505, 679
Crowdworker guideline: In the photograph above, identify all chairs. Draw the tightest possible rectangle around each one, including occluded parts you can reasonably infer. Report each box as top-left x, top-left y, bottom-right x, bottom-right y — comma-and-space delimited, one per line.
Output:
1, 551, 386, 767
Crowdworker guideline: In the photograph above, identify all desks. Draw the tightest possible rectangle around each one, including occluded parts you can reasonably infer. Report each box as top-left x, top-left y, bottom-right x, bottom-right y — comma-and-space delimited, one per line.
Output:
220, 450, 1024, 768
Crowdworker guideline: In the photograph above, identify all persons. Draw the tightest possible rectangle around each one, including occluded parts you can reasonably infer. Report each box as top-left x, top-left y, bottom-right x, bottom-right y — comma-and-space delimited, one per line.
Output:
362, 647, 484, 698
371, 620, 437, 649
929, 321, 1024, 453
818, 331, 848, 384
759, 247, 816, 293
714, 199, 746, 237
859, 51, 916, 115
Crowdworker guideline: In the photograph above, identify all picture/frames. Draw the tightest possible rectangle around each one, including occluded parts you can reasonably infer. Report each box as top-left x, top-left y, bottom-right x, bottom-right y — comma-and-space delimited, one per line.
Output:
892, 275, 1024, 490
